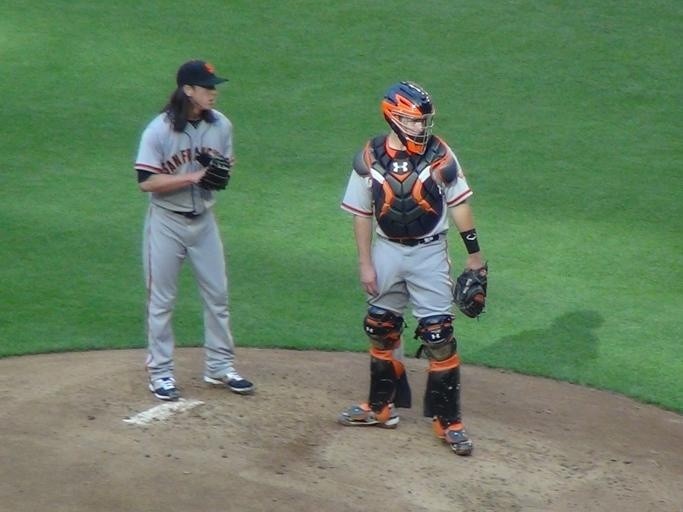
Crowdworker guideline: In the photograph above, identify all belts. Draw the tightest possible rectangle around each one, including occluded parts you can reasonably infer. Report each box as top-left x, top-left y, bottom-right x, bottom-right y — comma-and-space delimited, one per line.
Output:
377, 233, 440, 246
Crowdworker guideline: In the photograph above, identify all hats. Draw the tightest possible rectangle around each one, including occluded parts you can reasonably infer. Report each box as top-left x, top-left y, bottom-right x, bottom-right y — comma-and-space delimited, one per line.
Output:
176, 59, 229, 88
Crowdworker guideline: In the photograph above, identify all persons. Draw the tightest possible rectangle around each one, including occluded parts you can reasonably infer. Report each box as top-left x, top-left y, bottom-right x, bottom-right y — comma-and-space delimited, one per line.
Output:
339, 81, 487, 455
134, 60, 253, 400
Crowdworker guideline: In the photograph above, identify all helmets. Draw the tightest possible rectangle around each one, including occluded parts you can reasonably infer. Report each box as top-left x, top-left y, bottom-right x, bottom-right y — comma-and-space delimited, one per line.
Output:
381, 80, 435, 157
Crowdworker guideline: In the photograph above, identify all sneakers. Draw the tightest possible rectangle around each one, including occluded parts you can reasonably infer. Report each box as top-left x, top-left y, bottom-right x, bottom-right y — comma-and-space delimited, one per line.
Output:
338, 401, 400, 428
432, 414, 473, 456
203, 367, 254, 392
149, 375, 179, 400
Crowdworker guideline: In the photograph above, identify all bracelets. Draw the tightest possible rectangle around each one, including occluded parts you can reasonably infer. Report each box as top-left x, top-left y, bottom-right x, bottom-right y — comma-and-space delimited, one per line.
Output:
459, 227, 480, 254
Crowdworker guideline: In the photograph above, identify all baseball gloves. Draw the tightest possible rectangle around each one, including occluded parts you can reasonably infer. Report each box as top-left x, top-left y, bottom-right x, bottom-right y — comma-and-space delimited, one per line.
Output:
453, 265, 487, 317
195, 149, 230, 190
